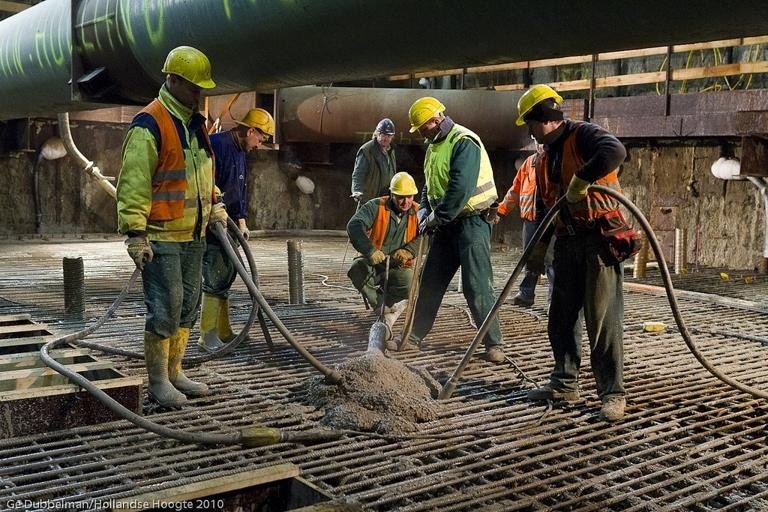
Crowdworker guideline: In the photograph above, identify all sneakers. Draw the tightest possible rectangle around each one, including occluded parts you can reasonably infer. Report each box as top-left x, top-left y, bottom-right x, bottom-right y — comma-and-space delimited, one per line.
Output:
486, 347, 507, 364
387, 334, 422, 352
503, 292, 535, 311
600, 392, 626, 421
527, 385, 579, 400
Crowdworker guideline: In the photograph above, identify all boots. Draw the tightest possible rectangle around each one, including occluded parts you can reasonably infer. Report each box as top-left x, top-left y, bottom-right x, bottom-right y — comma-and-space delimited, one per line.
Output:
170, 328, 208, 394
216, 297, 238, 344
197, 293, 226, 354
142, 332, 189, 408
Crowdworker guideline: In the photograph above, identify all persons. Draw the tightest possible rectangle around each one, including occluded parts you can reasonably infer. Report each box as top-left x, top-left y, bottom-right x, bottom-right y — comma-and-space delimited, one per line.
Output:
386, 97, 505, 362
346, 171, 422, 313
493, 134, 547, 307
351, 118, 397, 203
198, 107, 274, 352
516, 84, 642, 421
116, 44, 228, 406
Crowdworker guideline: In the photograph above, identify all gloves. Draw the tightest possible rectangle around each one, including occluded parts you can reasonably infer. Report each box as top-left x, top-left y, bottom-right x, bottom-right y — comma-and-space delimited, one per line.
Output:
240, 216, 250, 242
208, 206, 229, 228
413, 184, 428, 224
565, 172, 592, 206
416, 211, 438, 238
124, 237, 154, 269
369, 250, 386, 266
391, 248, 413, 267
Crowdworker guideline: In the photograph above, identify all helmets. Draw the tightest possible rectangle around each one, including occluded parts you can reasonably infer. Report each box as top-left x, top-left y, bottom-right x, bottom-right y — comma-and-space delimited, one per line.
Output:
388, 171, 419, 196
161, 46, 217, 90
376, 117, 396, 136
515, 84, 563, 128
234, 108, 276, 140
407, 96, 446, 135
